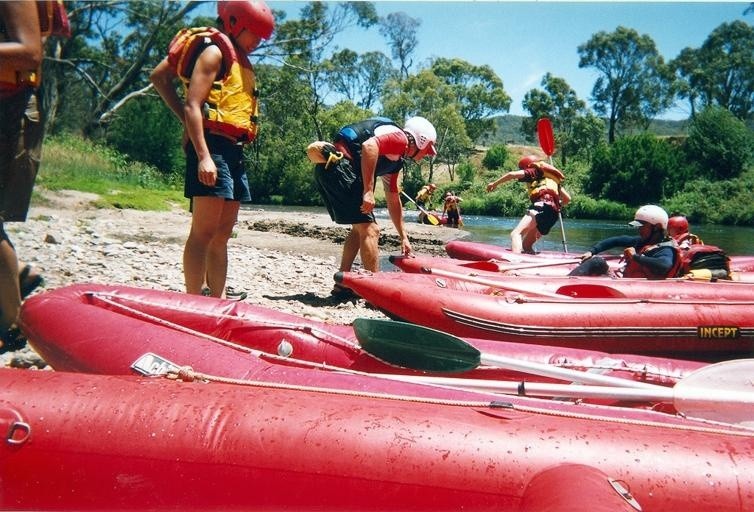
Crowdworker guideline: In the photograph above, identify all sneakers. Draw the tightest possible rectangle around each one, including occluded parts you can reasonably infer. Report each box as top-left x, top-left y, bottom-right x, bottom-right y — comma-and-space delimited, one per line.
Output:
224, 285, 248, 301
203, 287, 213, 298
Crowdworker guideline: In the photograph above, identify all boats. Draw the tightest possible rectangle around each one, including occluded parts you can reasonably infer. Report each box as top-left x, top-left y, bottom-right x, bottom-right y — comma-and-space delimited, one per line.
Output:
0, 365, 754, 512
389, 253, 754, 282
422, 210, 462, 226
445, 240, 754, 272
334, 270, 754, 356
18, 284, 754, 429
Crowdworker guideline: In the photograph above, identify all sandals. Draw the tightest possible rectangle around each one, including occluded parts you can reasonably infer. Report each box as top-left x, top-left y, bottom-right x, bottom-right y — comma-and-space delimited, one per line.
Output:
331, 284, 353, 298
18, 263, 45, 303
1, 321, 29, 357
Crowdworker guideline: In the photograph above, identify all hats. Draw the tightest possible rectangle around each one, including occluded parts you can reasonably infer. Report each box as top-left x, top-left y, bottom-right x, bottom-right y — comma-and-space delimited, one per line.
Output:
628, 220, 647, 227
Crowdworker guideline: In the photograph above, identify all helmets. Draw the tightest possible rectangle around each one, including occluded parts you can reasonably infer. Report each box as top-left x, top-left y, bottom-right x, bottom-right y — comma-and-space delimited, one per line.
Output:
630, 204, 669, 231
518, 155, 537, 169
217, 1, 275, 40
446, 192, 451, 196
668, 215, 689, 237
403, 115, 438, 154
429, 184, 437, 192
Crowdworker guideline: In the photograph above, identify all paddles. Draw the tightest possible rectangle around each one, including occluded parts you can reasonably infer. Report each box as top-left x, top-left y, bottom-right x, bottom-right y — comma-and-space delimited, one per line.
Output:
538, 117, 570, 255
400, 189, 440, 226
344, 358, 754, 432
419, 265, 627, 300
463, 254, 626, 273
352, 319, 672, 388
451, 190, 465, 227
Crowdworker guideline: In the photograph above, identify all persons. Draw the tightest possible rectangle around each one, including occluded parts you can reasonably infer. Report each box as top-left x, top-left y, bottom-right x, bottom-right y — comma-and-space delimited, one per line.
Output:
149, 1, 276, 297
486, 155, 572, 254
566, 204, 682, 278
668, 216, 704, 264
442, 192, 464, 228
415, 183, 436, 224
304, 114, 438, 298
0, 0, 52, 355
